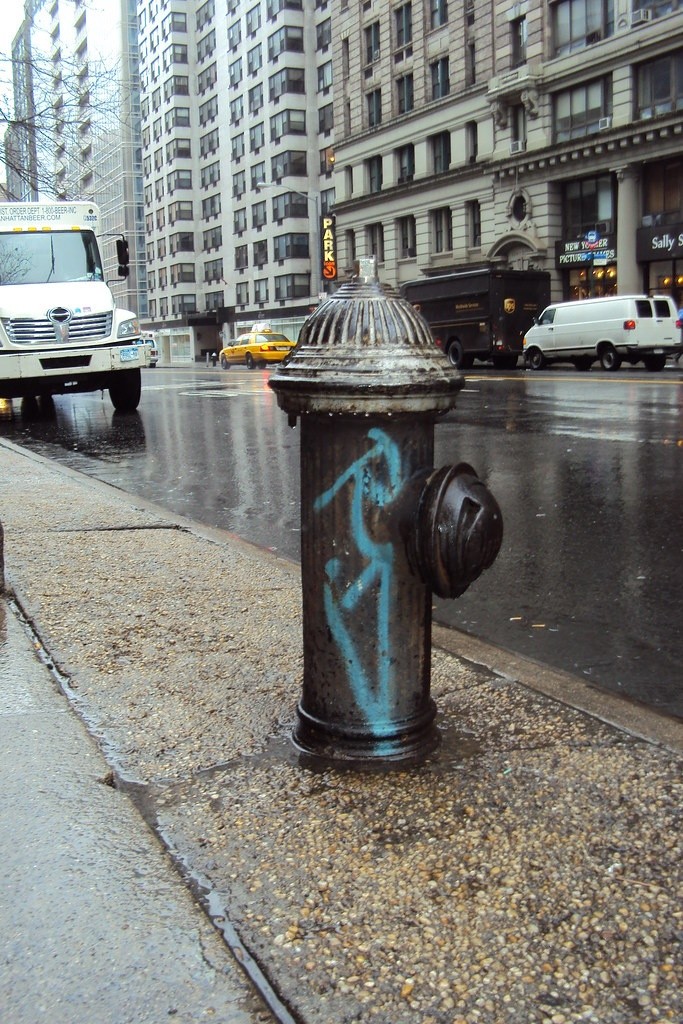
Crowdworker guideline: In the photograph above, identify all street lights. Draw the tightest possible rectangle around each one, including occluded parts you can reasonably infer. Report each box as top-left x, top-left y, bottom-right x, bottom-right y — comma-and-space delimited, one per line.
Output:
255, 181, 322, 307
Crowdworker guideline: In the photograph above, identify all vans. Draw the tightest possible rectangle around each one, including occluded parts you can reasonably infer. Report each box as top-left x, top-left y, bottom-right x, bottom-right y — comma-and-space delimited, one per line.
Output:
521, 292, 683, 372
132, 331, 159, 368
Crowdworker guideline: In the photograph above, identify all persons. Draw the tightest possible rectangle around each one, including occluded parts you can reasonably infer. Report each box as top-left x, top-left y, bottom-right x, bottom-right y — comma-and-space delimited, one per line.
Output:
542, 314, 551, 324
674, 300, 683, 364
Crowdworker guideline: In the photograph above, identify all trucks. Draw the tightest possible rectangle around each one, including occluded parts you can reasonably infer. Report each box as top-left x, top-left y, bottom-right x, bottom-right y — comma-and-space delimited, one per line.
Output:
0, 199, 142, 414
398, 267, 552, 371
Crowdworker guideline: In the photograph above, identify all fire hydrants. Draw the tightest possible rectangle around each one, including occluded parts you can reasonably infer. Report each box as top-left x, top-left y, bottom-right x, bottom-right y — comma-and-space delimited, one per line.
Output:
261, 256, 506, 777
210, 352, 217, 367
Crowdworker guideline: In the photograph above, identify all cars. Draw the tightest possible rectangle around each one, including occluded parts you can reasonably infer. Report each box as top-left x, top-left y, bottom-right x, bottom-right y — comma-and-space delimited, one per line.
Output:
217, 321, 297, 371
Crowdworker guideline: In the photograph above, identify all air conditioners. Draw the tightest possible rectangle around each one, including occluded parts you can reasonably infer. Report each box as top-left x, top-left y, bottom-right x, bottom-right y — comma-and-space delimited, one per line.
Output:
631, 8, 653, 28
599, 117, 611, 129
595, 219, 615, 236
509, 141, 525, 154
